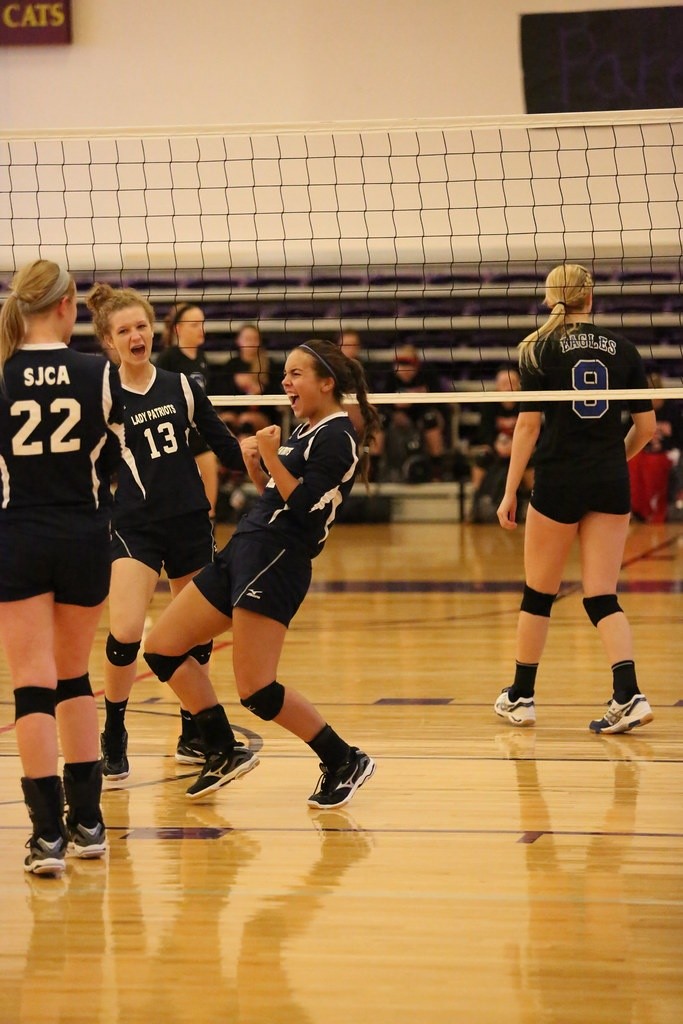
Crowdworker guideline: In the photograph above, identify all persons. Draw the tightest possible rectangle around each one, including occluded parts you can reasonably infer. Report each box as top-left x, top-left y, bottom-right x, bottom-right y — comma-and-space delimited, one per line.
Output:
621, 376, 683, 523
0, 261, 126, 875
85, 286, 248, 779
143, 341, 380, 809
154, 304, 544, 549
495, 263, 655, 734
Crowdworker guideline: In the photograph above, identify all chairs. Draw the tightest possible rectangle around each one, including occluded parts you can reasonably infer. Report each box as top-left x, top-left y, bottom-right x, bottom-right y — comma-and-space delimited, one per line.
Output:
0, 267, 683, 519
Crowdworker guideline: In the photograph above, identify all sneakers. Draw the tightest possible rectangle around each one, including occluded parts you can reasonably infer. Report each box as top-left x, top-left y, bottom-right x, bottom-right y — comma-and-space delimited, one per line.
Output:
306, 746, 377, 809
494, 686, 536, 727
185, 741, 261, 800
100, 729, 131, 780
174, 734, 207, 765
23, 832, 66, 874
64, 818, 106, 858
588, 693, 656, 734
